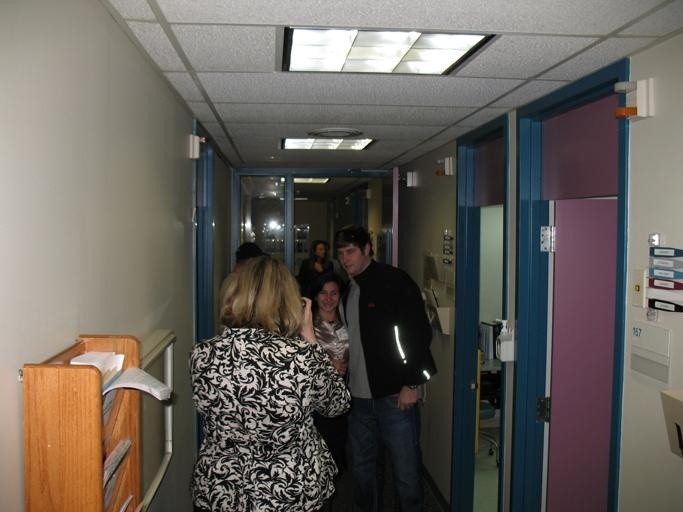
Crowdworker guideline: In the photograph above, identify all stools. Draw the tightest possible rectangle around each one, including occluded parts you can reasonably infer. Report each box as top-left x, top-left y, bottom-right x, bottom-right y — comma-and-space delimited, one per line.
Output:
479, 402, 500, 466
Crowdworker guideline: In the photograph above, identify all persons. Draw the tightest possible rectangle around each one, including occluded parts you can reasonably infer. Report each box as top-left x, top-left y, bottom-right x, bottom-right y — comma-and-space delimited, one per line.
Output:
186, 255, 353, 512
237, 242, 263, 262
296, 240, 350, 296
309, 273, 350, 511
333, 225, 438, 511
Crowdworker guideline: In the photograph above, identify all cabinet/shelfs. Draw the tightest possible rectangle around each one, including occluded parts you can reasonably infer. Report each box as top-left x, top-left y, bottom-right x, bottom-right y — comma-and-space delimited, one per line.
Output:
23, 334, 143, 512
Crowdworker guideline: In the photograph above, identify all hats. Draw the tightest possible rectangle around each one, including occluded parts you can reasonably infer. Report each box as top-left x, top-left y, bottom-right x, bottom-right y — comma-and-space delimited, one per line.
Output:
236, 242, 270, 260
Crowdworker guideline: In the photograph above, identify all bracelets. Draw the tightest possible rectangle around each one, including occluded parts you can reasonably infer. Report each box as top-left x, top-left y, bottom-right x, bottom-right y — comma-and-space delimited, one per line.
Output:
409, 384, 418, 389
